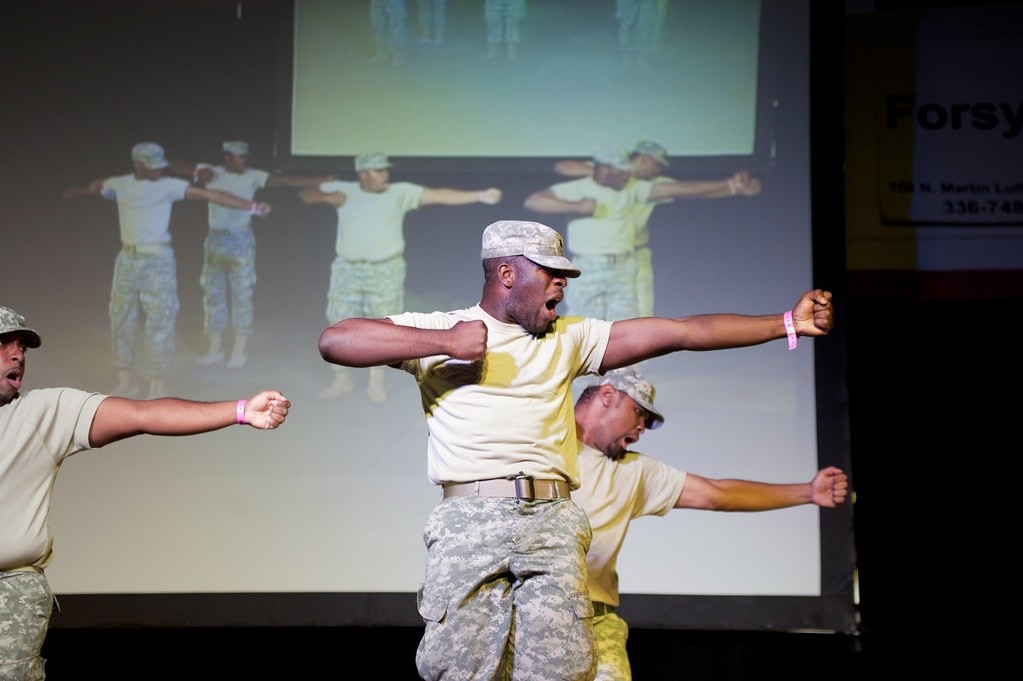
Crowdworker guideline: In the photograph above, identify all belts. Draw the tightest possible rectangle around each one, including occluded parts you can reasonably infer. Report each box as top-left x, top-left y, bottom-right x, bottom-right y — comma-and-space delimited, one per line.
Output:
343, 254, 398, 265
7, 566, 41, 572
209, 224, 249, 234
634, 244, 645, 250
592, 601, 615, 616
123, 243, 168, 254
586, 253, 628, 264
443, 476, 571, 502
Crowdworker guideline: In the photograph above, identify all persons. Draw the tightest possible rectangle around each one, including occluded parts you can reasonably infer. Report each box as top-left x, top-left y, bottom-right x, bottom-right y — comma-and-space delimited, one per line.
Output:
366, 0, 407, 68
300, 153, 500, 405
319, 221, 831, 681
485, 0, 527, 61
66, 141, 338, 400
524, 142, 760, 318
493, 366, 847, 681
616, 0, 669, 75
0, 306, 291, 681
418, 0, 446, 45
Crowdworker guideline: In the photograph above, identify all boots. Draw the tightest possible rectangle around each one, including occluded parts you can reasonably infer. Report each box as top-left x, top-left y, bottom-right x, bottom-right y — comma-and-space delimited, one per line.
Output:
319, 376, 353, 399
146, 374, 169, 399
111, 371, 140, 397
228, 336, 248, 369
197, 333, 223, 366
367, 372, 385, 404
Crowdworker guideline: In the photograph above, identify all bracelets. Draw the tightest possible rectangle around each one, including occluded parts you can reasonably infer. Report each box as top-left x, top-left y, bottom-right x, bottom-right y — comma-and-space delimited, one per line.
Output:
729, 177, 737, 196
237, 400, 246, 425
194, 169, 199, 182
252, 201, 257, 215
783, 310, 800, 350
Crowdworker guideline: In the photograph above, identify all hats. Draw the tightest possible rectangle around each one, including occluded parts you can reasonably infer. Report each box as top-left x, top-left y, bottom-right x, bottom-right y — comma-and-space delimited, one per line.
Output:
355, 152, 392, 172
589, 367, 666, 430
636, 140, 669, 166
0, 306, 42, 349
223, 141, 248, 155
131, 142, 170, 170
480, 219, 582, 278
593, 145, 636, 172
